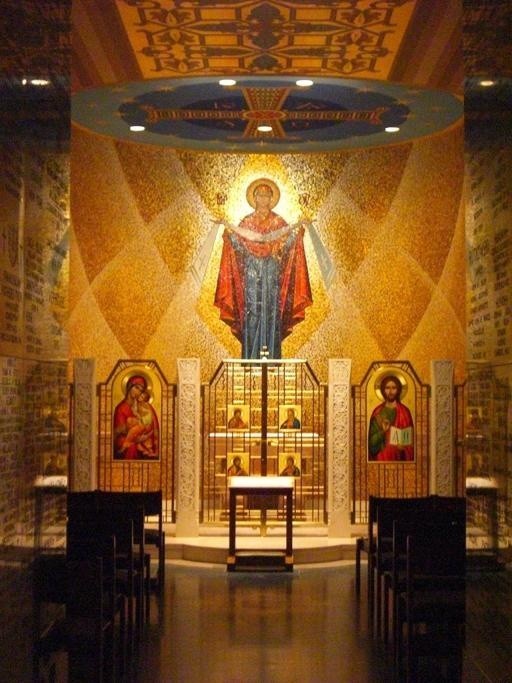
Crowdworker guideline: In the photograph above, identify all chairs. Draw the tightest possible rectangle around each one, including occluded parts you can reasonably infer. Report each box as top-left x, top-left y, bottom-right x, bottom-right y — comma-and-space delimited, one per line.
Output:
356, 495, 467, 683
24, 490, 165, 682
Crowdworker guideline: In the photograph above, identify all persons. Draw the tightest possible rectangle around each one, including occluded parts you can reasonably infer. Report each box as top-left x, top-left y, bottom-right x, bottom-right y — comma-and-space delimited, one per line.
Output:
210, 184, 318, 368
228, 455, 247, 476
279, 406, 299, 429
112, 375, 159, 458
369, 375, 415, 459
227, 408, 247, 428
277, 454, 302, 477
118, 391, 156, 454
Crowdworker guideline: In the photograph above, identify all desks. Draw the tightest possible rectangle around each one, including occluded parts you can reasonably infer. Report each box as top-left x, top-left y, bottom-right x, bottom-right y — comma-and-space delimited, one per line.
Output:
227, 475, 295, 573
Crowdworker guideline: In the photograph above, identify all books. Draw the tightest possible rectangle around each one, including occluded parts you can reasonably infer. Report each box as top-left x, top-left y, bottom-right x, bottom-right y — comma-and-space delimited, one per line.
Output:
387, 425, 413, 447
